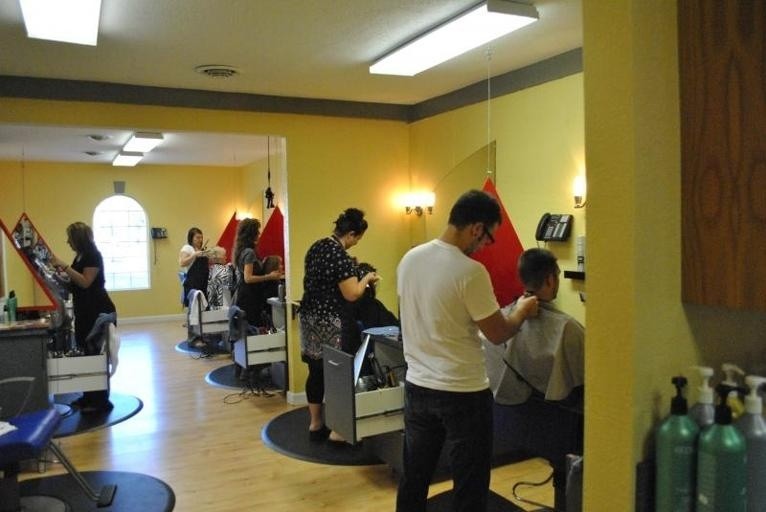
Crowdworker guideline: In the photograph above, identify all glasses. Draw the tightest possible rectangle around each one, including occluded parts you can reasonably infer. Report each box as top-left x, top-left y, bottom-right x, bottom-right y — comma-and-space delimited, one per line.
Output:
483, 226, 494, 245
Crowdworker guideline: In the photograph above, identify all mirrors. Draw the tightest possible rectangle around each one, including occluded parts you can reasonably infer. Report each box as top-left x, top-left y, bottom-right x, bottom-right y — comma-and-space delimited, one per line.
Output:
247, 191, 263, 227
425, 140, 496, 257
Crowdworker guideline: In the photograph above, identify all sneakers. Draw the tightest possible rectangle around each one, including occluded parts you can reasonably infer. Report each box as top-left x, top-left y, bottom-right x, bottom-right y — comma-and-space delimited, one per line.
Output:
310, 425, 345, 445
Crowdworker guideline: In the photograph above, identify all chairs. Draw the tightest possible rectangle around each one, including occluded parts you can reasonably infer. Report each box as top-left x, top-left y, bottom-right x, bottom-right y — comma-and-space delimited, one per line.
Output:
512, 387, 583, 512
0, 376, 117, 512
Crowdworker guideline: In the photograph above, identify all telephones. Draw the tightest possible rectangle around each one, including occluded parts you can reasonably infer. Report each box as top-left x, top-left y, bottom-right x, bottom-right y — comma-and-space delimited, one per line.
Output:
535, 213, 572, 241
151, 226, 168, 240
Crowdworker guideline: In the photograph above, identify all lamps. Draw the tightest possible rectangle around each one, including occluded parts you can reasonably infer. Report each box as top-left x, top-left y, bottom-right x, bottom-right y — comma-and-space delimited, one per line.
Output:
19, 0, 102, 47
112, 154, 144, 167
368, 0, 549, 78
122, 132, 164, 153
571, 160, 587, 209
392, 189, 436, 215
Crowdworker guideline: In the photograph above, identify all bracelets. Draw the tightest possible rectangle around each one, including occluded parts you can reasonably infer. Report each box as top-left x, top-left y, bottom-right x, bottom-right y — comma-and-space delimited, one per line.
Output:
63, 264, 70, 271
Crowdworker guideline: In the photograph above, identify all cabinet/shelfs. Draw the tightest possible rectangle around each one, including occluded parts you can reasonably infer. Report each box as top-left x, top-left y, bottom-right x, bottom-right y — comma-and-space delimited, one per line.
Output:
0, 313, 110, 473
229, 297, 288, 385
188, 285, 232, 353
322, 328, 406, 481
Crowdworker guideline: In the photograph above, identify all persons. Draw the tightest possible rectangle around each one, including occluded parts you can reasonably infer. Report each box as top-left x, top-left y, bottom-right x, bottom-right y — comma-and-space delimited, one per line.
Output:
262, 255, 283, 275
207, 245, 227, 265
52, 222, 116, 413
230, 216, 285, 381
394, 188, 540, 511
299, 207, 382, 443
516, 246, 562, 303
359, 261, 377, 298
179, 227, 208, 348
